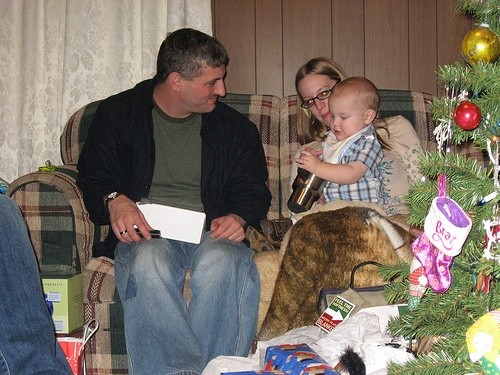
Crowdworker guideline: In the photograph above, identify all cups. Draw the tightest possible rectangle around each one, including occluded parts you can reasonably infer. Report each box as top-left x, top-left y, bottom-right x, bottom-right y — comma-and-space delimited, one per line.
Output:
287, 164, 327, 211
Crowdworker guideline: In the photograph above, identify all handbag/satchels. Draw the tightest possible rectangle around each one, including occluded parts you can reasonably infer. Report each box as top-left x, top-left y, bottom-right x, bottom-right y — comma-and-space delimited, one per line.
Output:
318, 262, 396, 318
56, 318, 98, 375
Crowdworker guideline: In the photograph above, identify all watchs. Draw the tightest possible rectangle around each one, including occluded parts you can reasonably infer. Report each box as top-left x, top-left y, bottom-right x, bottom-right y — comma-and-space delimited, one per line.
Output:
106, 191, 121, 213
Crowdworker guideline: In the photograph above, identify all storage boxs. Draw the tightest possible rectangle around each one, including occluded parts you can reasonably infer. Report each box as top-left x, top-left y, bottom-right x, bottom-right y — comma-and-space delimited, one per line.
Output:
40, 273, 85, 336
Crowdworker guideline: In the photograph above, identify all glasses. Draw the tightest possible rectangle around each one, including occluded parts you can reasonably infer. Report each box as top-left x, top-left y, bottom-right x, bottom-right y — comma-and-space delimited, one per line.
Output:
301, 80, 339, 109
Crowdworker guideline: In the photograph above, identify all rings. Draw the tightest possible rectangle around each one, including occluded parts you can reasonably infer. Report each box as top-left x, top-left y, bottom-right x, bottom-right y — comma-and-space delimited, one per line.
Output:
120, 229, 127, 235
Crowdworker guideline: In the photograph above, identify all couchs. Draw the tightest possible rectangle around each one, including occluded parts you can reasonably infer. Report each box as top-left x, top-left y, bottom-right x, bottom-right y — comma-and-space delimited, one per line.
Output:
5, 90, 439, 375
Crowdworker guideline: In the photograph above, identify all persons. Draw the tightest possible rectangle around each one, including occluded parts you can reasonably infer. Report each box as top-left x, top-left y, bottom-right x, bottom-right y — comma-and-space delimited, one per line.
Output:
295, 59, 426, 216
296, 76, 391, 206
77, 28, 274, 375
0, 195, 76, 375
